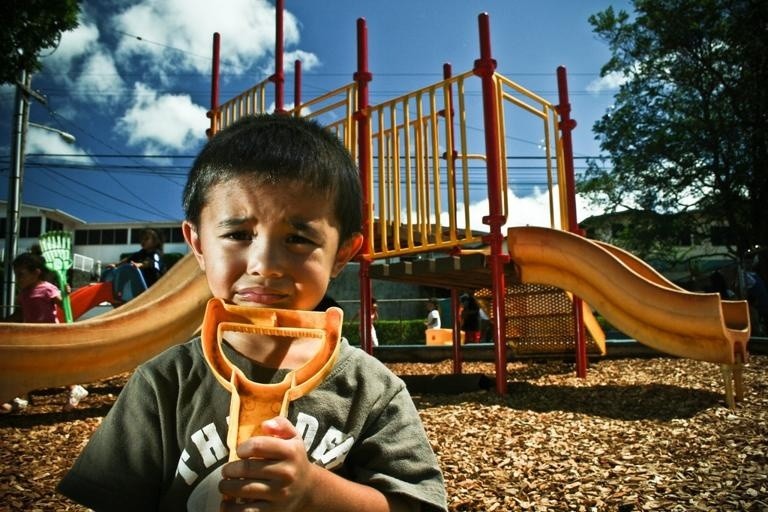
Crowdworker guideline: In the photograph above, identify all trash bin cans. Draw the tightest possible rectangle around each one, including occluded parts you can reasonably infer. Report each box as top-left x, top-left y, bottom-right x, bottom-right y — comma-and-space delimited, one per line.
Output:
425, 330, 465, 345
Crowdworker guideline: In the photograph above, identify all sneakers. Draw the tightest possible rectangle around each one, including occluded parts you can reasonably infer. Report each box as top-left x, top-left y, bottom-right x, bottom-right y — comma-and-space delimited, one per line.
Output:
62, 384, 89, 412
9, 397, 28, 415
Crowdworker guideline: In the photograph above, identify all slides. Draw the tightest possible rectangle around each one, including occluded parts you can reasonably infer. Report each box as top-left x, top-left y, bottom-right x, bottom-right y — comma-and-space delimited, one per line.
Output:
0, 251, 213, 406
56, 279, 112, 323
509, 228, 751, 363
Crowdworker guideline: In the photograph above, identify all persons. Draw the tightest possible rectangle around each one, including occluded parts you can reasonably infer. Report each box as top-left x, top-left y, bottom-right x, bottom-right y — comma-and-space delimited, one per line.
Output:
457, 292, 495, 345
51, 108, 453, 512
346, 294, 381, 347
0, 252, 90, 410
422, 298, 443, 330
105, 228, 168, 286
